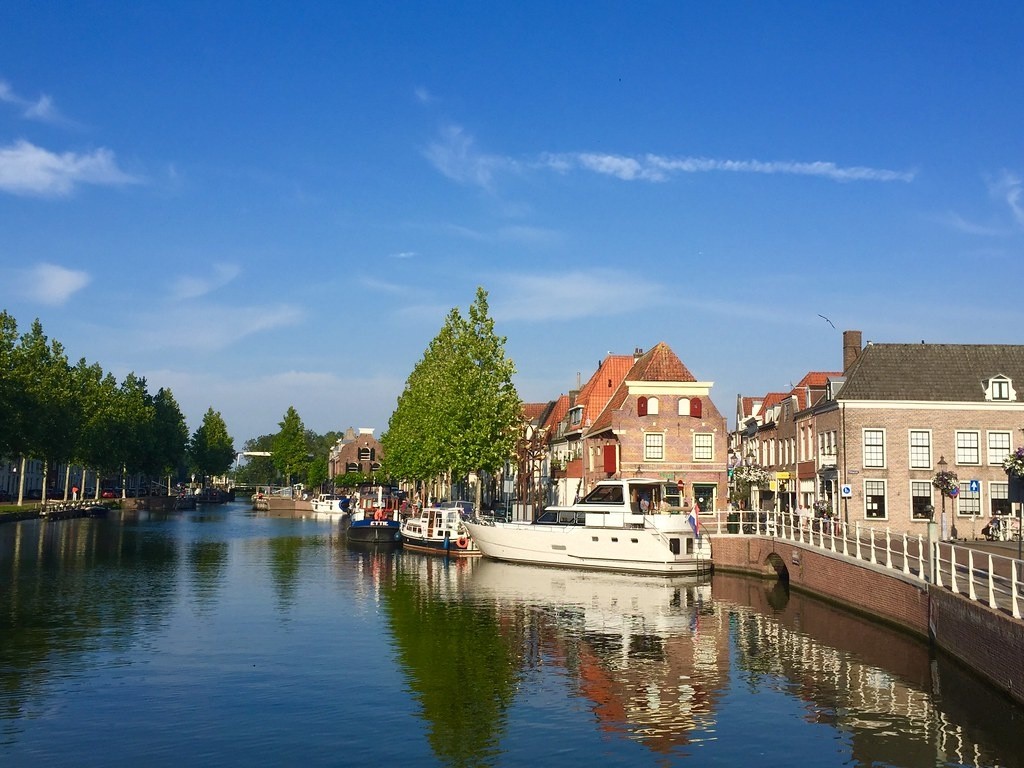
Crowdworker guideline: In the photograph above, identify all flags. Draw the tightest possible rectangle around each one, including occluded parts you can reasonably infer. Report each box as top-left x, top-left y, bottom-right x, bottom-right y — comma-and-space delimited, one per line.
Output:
687, 503, 699, 538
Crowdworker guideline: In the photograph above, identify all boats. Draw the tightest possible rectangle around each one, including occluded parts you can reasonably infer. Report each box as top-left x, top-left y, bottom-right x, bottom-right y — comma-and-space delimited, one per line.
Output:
192, 473, 238, 503
400, 507, 485, 556
310, 482, 400, 543
459, 477, 714, 576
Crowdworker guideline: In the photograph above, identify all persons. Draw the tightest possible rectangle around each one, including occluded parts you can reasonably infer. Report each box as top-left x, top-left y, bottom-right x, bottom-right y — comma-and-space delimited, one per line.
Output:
784, 504, 790, 525
400, 497, 422, 518
794, 505, 810, 528
72, 485, 78, 501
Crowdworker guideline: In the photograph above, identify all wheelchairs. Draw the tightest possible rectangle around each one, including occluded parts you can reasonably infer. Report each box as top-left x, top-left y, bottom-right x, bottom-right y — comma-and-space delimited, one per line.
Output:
985, 522, 1000, 542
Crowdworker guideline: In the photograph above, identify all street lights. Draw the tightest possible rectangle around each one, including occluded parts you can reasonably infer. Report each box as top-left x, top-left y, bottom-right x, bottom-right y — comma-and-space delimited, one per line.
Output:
937, 455, 949, 540
744, 449, 757, 534
11, 465, 18, 505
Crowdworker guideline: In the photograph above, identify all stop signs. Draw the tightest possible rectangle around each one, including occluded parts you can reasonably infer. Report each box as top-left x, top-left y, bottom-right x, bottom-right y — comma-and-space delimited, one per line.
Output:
678, 480, 684, 491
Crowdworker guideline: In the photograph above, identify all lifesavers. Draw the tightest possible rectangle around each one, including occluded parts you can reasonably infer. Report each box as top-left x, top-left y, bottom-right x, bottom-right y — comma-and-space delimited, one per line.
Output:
456, 536, 468, 548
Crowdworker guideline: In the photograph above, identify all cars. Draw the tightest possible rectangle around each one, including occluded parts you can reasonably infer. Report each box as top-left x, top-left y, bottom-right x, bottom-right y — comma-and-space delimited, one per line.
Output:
29, 487, 159, 500
